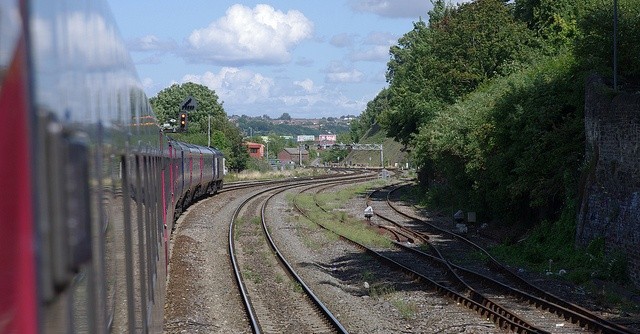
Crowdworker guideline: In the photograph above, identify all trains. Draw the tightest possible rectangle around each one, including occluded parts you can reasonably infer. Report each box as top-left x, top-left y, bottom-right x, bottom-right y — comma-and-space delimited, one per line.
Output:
0, 0, 223, 334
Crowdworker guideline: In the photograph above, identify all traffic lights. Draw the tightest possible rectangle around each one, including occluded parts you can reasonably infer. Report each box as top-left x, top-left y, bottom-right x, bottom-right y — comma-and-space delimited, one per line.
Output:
180, 113, 187, 129
